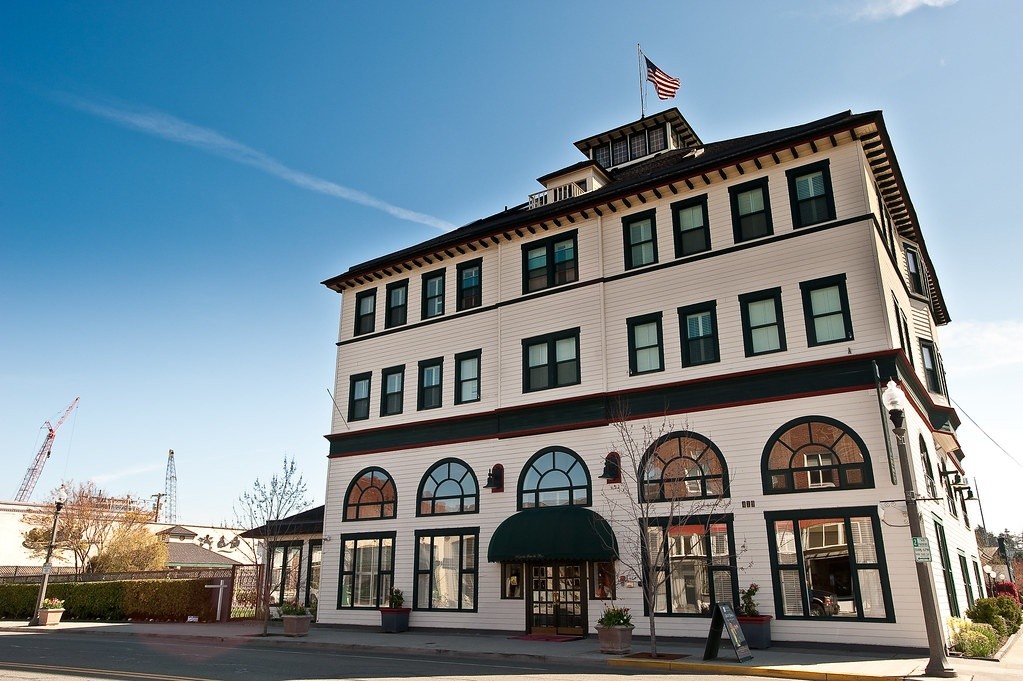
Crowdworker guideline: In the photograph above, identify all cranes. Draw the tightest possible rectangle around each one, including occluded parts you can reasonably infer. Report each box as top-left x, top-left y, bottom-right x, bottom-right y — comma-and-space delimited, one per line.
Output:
13, 396, 80, 498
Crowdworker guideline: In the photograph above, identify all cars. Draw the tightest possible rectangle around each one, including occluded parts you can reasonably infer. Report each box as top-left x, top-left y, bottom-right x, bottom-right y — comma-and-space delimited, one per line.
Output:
262, 584, 319, 607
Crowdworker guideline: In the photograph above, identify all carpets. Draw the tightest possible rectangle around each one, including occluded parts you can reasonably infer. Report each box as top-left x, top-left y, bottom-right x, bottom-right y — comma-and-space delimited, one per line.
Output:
507, 634, 581, 642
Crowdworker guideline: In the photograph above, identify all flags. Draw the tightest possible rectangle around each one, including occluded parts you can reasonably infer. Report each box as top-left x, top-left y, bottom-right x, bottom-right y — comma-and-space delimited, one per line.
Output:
642, 53, 681, 100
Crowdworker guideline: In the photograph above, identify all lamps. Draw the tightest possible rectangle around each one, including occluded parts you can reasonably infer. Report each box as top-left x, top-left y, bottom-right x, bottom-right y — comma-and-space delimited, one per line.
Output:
938, 462, 965, 487
952, 480, 979, 501
483, 468, 500, 488
598, 456, 618, 480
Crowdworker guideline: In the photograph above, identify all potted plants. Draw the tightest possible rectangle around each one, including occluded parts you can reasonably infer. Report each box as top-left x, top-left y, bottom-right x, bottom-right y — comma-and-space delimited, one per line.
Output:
378, 586, 411, 633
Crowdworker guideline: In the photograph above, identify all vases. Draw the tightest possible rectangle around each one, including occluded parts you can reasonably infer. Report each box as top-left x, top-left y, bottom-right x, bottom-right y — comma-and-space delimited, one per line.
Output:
38, 609, 66, 625
736, 615, 772, 649
594, 624, 635, 655
282, 615, 312, 636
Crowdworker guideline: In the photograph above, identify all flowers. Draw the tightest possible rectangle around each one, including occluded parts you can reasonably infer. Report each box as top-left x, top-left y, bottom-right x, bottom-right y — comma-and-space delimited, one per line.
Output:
41, 598, 65, 609
279, 602, 307, 615
597, 608, 634, 629
735, 583, 761, 617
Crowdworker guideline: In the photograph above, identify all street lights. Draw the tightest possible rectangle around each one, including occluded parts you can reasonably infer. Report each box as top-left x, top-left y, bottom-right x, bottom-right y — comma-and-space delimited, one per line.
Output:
982, 565, 1005, 597
28, 484, 68, 626
881, 376, 957, 677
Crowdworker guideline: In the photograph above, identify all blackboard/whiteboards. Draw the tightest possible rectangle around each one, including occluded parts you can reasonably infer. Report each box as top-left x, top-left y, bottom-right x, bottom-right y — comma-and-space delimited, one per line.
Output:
703, 602, 755, 662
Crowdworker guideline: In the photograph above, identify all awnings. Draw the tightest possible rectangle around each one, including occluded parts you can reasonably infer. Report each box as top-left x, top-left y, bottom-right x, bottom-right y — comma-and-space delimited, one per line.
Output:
487, 505, 619, 564
